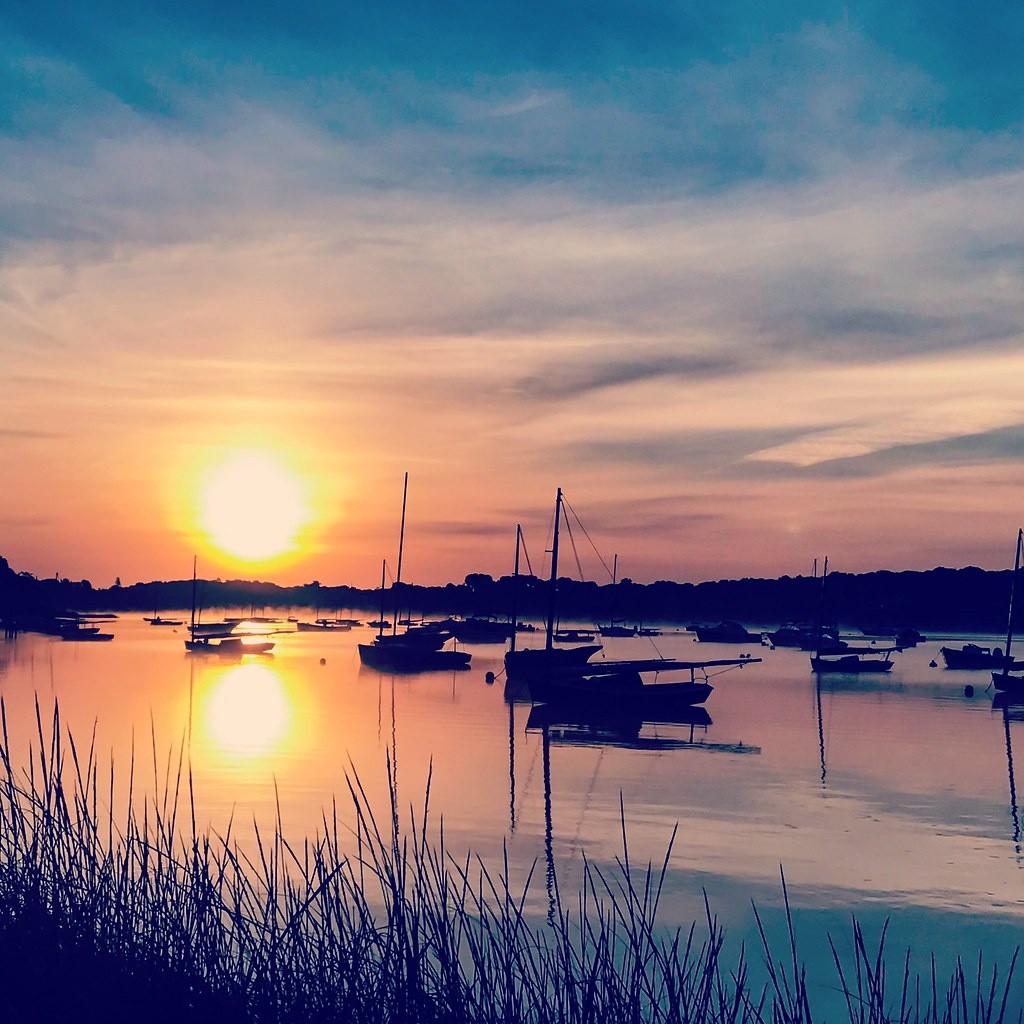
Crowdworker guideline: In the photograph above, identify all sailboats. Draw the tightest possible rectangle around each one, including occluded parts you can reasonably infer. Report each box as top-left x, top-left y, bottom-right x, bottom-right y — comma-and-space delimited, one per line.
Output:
144, 473, 1024, 733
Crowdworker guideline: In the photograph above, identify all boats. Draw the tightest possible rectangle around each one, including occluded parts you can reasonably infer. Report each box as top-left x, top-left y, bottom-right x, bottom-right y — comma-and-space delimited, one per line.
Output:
60, 610, 120, 641
